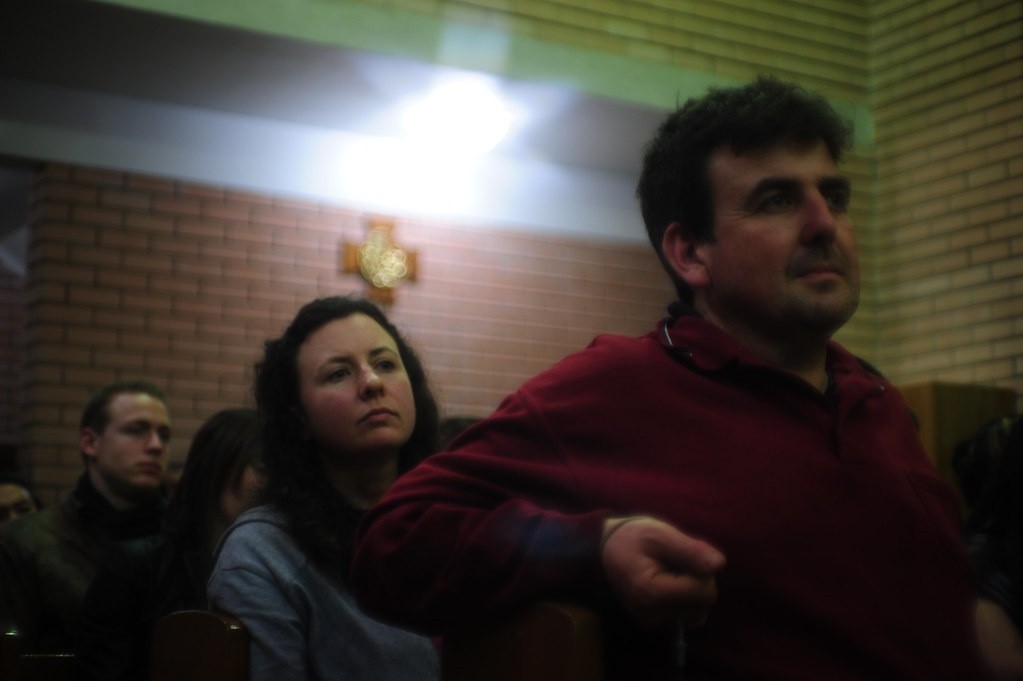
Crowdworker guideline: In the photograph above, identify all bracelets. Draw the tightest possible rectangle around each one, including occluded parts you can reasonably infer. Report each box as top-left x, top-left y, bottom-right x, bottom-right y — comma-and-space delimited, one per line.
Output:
598, 515, 654, 558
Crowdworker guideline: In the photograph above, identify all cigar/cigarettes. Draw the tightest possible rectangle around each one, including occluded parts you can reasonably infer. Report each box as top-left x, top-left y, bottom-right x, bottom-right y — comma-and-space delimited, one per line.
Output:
677, 614, 685, 667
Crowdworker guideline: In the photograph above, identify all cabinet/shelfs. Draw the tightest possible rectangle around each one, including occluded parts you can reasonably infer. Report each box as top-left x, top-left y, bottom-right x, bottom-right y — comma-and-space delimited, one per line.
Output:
900, 382, 1017, 464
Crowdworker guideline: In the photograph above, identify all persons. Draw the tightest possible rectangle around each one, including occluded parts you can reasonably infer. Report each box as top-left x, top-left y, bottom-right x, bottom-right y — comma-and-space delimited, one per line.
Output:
0, 379, 261, 679
204, 294, 444, 680
952, 413, 1022, 623
349, 73, 1023, 680
